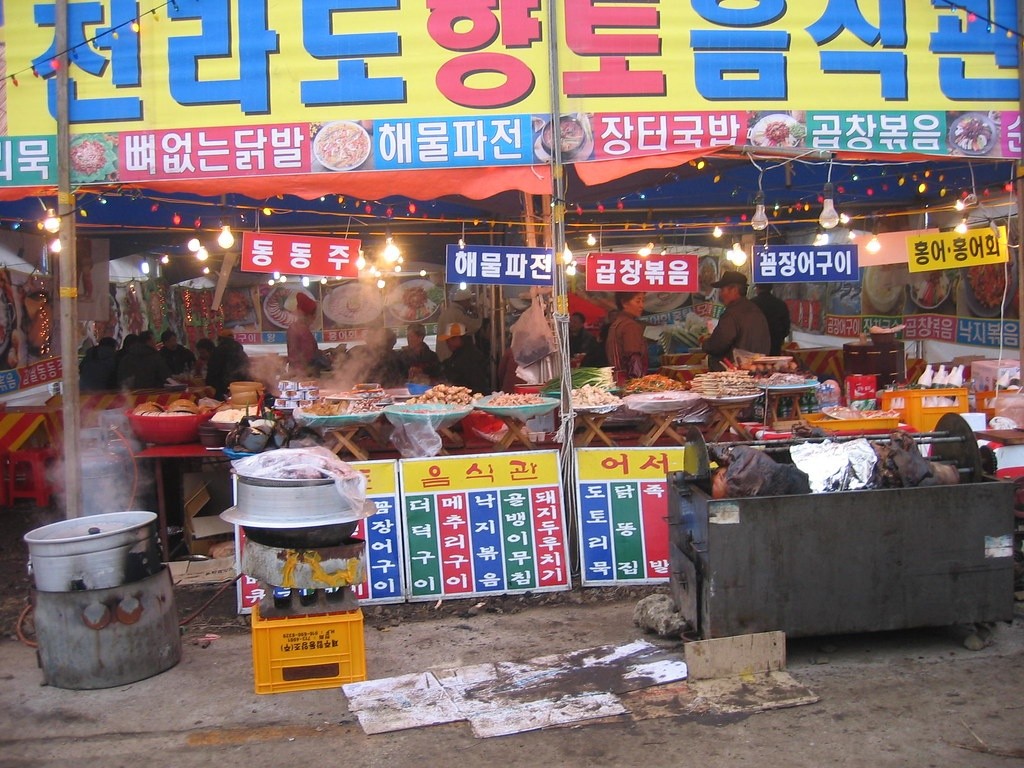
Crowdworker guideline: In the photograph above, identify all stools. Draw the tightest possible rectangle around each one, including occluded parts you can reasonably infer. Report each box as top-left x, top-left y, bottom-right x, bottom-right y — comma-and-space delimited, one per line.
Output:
8, 444, 58, 508
637, 410, 686, 447
764, 388, 810, 429
492, 414, 537, 452
321, 423, 370, 461
576, 412, 619, 447
705, 401, 753, 443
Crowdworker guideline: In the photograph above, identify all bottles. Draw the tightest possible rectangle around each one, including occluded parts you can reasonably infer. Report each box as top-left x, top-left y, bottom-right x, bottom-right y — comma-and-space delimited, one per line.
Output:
751, 389, 819, 423
882, 362, 964, 406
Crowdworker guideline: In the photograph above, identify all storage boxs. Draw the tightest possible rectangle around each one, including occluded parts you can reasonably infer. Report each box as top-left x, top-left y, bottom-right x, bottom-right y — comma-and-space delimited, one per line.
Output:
182, 471, 234, 555
970, 358, 1021, 392
976, 389, 1019, 421
881, 389, 968, 434
251, 601, 368, 695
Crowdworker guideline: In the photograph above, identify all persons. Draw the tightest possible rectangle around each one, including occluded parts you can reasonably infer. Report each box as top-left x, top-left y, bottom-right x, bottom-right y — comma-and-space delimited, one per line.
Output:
701, 271, 789, 373
78, 328, 252, 401
285, 292, 491, 396
500, 347, 526, 395
570, 291, 648, 387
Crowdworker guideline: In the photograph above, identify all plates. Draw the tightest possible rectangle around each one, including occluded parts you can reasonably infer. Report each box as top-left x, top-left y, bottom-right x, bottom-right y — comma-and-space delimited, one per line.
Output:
642, 291, 690, 315
387, 279, 440, 322
322, 283, 383, 325
961, 243, 1018, 318
262, 284, 316, 329
822, 407, 866, 421
696, 258, 716, 300
949, 112, 997, 156
313, 121, 371, 171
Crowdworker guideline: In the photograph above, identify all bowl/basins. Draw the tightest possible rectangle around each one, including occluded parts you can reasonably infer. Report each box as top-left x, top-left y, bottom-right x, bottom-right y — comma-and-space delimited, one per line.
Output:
124, 382, 265, 446
541, 116, 586, 160
908, 271, 951, 308
230, 466, 350, 487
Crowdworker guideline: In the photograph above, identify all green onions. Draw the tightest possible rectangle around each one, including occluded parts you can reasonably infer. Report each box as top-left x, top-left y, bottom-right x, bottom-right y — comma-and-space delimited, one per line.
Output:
541, 365, 618, 391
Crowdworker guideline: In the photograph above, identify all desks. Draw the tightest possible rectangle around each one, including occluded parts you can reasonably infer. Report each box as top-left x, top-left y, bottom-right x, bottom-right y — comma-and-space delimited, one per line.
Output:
657, 365, 709, 390
662, 354, 706, 367
135, 443, 226, 563
0, 384, 216, 507
784, 346, 845, 386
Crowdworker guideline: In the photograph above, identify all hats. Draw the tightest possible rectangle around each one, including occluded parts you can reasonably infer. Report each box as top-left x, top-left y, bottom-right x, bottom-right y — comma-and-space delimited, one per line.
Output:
437, 323, 468, 340
711, 271, 747, 288
296, 292, 318, 313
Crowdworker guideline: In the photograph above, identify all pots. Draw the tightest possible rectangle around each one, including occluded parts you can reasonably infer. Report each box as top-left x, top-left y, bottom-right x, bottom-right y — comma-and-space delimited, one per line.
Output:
24, 510, 158, 592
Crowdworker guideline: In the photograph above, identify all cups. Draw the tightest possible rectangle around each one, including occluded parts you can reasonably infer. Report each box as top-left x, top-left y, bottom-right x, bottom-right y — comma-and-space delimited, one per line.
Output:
865, 266, 902, 313
